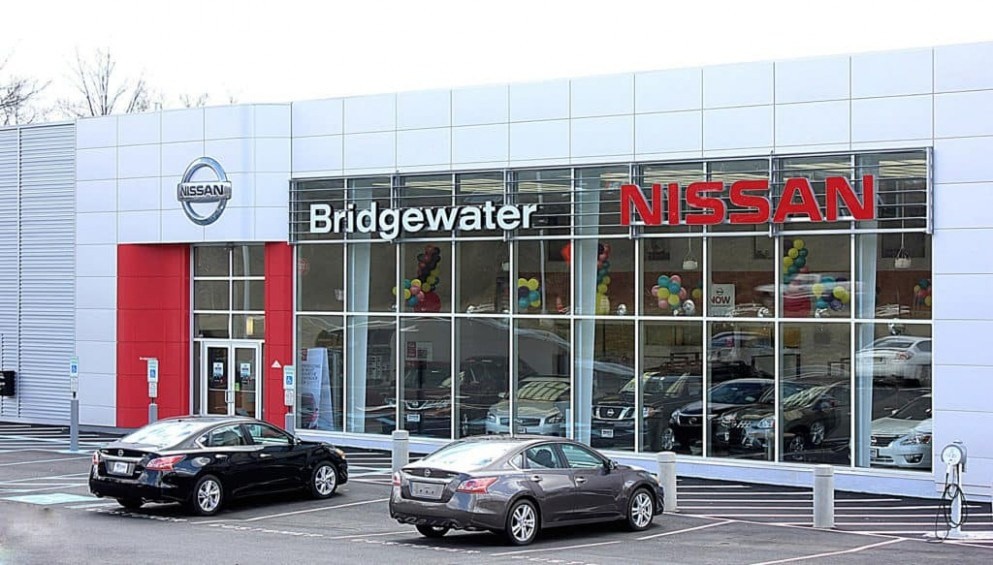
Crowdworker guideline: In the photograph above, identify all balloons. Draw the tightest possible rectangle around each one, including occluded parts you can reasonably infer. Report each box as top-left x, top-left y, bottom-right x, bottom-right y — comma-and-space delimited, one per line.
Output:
391, 246, 442, 312
652, 275, 701, 314
517, 277, 541, 313
596, 243, 611, 294
914, 279, 932, 306
812, 277, 850, 310
784, 239, 808, 292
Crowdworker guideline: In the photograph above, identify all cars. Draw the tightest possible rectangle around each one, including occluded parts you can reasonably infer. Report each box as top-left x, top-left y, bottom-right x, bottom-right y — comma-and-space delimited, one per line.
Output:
856, 336, 931, 387
388, 434, 664, 547
710, 331, 801, 374
88, 413, 349, 517
716, 376, 909, 461
754, 273, 866, 310
468, 353, 537, 393
670, 378, 800, 454
849, 395, 932, 469
383, 360, 518, 440
486, 376, 570, 436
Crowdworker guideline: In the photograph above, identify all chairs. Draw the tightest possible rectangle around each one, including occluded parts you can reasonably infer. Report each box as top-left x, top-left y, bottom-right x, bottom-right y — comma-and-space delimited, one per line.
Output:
521, 448, 555, 468
221, 431, 240, 446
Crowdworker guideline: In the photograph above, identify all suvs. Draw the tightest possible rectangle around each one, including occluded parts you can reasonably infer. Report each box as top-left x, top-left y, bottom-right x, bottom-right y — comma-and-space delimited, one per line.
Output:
591, 359, 779, 452
